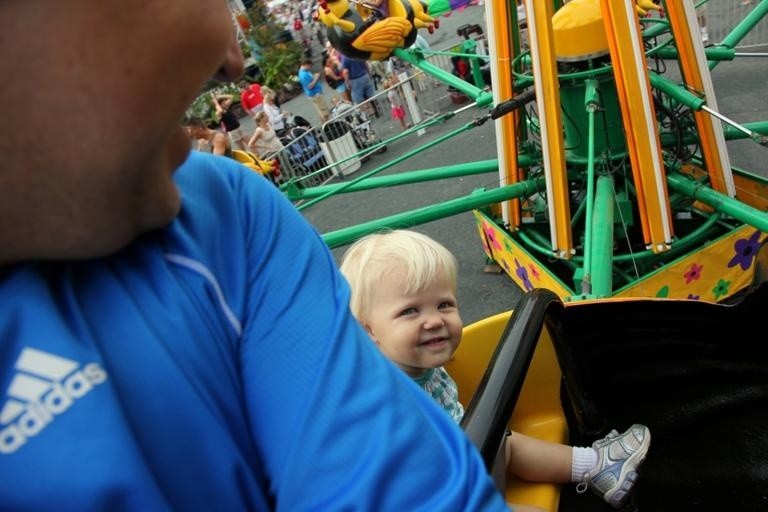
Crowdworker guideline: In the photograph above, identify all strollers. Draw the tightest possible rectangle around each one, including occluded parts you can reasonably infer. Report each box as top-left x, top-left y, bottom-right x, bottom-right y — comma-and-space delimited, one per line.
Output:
266, 102, 386, 191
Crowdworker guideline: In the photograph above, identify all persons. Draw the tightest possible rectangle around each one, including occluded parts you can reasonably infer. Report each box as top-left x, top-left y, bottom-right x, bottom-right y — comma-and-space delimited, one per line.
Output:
337, 226, 653, 510
182, 0, 439, 190
0, 0, 514, 512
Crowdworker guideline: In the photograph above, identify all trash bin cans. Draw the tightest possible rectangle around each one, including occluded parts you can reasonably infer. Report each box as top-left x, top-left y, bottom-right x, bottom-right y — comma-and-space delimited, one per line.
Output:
318, 120, 362, 177
456, 24, 488, 55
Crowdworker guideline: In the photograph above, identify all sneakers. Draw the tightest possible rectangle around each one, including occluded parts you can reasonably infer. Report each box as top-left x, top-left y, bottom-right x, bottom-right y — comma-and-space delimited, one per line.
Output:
576, 424, 651, 506
